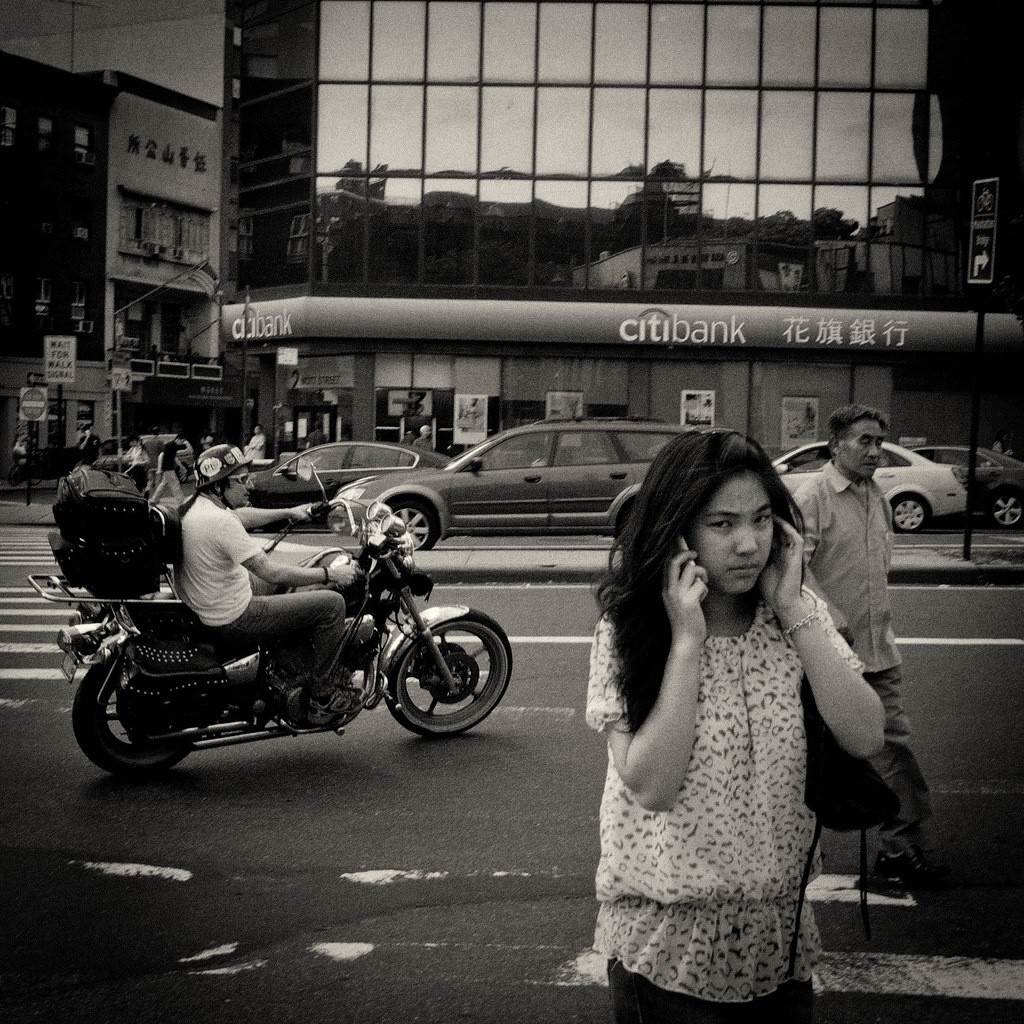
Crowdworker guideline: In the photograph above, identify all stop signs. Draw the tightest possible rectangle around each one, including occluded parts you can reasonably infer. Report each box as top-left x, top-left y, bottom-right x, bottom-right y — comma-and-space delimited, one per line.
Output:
21, 388, 46, 420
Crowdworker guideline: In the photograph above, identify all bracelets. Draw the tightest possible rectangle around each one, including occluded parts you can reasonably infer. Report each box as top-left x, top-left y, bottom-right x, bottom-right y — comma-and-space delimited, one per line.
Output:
783, 610, 820, 639
321, 565, 330, 584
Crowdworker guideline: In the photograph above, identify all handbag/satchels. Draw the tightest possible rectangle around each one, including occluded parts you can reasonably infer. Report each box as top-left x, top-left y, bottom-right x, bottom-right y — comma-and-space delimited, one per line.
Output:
799, 676, 901, 830
51, 468, 160, 600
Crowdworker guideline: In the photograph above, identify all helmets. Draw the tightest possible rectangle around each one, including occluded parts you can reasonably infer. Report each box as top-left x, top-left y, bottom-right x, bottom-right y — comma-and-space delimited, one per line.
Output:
193, 443, 253, 487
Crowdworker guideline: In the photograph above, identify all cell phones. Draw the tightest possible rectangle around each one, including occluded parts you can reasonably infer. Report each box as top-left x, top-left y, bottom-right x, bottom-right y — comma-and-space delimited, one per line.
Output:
672, 536, 709, 603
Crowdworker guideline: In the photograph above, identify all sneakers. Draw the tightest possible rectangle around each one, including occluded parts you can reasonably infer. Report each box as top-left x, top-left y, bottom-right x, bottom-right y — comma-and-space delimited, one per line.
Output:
310, 689, 368, 723
877, 846, 961, 891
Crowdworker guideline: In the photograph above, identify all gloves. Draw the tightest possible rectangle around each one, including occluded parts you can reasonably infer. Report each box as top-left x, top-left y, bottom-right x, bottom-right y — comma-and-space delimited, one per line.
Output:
333, 555, 357, 586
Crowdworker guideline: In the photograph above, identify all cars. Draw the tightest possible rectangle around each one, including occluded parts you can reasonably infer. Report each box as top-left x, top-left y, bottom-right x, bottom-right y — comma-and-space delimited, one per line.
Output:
242, 441, 449, 530
336, 415, 708, 550
909, 444, 1024, 529
137, 432, 195, 482
771, 441, 968, 534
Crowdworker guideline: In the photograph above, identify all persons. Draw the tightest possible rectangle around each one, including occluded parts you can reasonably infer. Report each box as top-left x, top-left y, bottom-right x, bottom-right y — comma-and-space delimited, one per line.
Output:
143, 425, 164, 499
173, 442, 366, 724
303, 420, 328, 449
120, 434, 150, 497
244, 424, 267, 460
78, 420, 105, 469
201, 426, 214, 451
993, 430, 1013, 457
149, 433, 188, 507
584, 424, 889, 1024
401, 423, 435, 452
789, 402, 954, 892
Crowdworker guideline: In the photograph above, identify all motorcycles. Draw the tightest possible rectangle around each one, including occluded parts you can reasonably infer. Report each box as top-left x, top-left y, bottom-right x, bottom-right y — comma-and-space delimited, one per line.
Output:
27, 456, 513, 776
